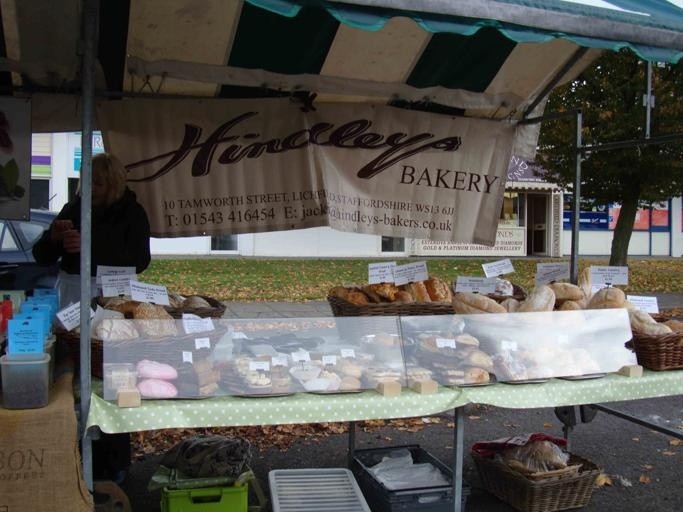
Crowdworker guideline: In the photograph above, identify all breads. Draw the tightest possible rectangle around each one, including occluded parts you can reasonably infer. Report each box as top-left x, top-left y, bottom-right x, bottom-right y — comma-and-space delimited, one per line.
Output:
70, 275, 683, 399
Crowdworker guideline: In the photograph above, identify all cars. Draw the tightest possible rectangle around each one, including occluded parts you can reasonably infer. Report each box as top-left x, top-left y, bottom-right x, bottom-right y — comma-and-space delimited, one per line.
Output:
0, 208, 61, 291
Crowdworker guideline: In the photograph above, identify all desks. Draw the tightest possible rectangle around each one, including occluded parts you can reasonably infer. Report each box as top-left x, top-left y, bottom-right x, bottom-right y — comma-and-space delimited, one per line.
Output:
90, 366, 682, 512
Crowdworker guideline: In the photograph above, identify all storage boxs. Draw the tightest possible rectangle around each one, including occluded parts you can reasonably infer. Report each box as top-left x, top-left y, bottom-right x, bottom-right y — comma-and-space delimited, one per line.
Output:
0, 331, 57, 410
266, 463, 374, 512
346, 443, 472, 512
155, 460, 253, 511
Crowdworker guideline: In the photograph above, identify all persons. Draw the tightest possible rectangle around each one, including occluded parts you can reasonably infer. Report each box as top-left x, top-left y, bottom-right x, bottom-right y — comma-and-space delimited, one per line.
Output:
31, 153, 153, 484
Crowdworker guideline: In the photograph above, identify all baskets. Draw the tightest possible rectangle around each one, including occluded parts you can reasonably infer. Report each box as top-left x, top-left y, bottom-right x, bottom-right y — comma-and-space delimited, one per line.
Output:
328, 281, 527, 371
328, 277, 683, 371
59, 294, 227, 379
630, 307, 682, 370
472, 446, 600, 511
